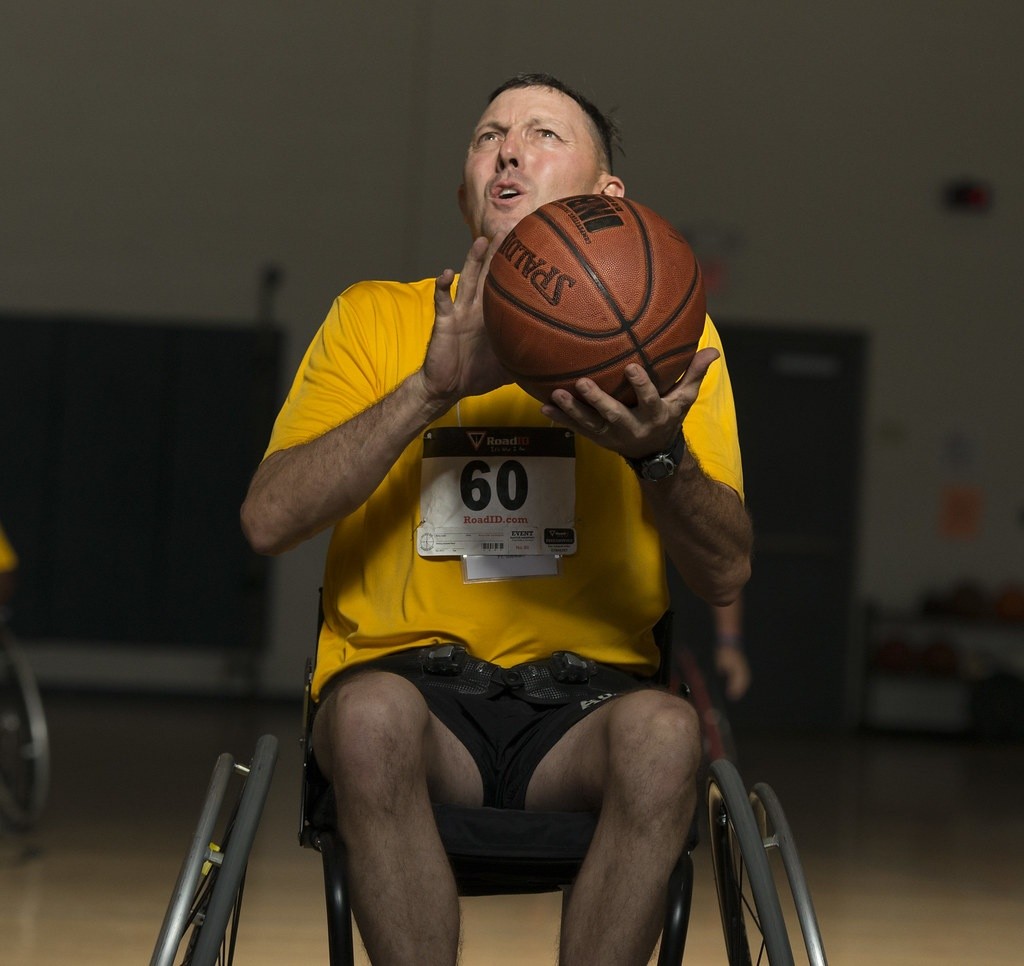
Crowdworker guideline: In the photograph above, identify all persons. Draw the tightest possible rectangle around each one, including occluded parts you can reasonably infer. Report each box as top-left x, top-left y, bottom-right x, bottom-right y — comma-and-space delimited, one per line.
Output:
234, 74, 757, 966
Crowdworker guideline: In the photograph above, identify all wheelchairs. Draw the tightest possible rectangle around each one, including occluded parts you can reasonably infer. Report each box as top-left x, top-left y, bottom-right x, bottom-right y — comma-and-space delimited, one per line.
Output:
1, 604, 53, 830
149, 586, 826, 966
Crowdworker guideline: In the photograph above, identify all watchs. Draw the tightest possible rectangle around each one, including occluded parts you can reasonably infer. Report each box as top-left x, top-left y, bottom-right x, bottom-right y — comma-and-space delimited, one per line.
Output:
623, 430, 686, 487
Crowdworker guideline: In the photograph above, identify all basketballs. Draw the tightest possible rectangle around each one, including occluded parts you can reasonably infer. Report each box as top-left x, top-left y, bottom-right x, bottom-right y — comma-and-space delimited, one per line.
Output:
482, 193, 707, 408
